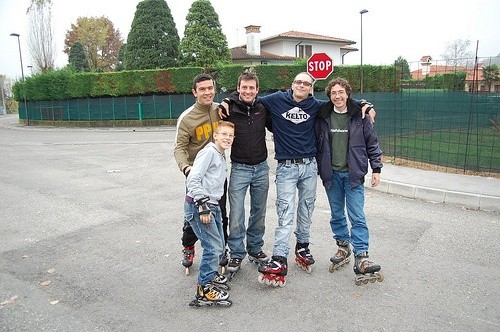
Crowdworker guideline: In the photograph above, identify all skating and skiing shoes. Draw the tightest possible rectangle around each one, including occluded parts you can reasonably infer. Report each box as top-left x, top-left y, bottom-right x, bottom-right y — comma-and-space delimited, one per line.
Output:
295, 242, 316, 272
181, 245, 195, 276
257, 255, 289, 287
248, 249, 270, 265
328, 243, 353, 273
353, 252, 385, 284
220, 254, 233, 273
214, 274, 232, 291
189, 284, 235, 308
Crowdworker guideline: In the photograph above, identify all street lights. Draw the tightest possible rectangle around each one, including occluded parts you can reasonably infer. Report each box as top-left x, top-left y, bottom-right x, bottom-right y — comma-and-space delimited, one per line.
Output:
10, 34, 29, 125
360, 9, 368, 94
28, 66, 32, 76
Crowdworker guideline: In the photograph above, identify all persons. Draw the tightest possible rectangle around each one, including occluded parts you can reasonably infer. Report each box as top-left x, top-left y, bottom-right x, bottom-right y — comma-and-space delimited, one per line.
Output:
172, 73, 228, 276
271, 78, 384, 286
217, 71, 376, 287
184, 121, 235, 306
218, 72, 274, 280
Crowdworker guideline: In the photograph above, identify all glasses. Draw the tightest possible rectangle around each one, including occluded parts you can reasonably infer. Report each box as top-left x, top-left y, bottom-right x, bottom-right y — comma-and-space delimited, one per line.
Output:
217, 132, 236, 139
331, 90, 345, 95
293, 80, 315, 87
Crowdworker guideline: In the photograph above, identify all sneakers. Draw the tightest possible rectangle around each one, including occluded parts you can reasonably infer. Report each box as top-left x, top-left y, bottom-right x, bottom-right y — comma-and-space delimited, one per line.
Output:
228, 256, 242, 277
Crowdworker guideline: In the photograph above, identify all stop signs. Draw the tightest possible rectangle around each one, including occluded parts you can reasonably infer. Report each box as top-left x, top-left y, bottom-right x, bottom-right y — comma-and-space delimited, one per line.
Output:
307, 52, 334, 80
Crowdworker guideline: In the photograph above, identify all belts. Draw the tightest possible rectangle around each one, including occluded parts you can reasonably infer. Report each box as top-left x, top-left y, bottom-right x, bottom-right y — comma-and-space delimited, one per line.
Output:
277, 158, 313, 164
234, 161, 264, 165
